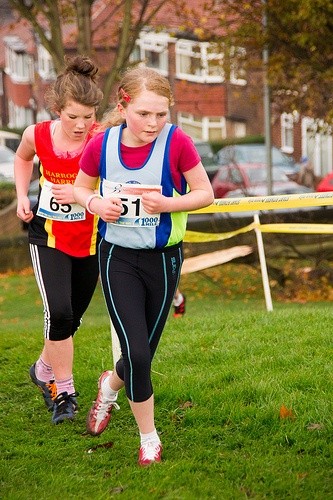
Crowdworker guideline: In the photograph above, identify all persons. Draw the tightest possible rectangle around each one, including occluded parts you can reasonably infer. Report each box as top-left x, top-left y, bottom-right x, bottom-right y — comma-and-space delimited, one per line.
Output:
14, 55, 107, 423
72, 66, 215, 467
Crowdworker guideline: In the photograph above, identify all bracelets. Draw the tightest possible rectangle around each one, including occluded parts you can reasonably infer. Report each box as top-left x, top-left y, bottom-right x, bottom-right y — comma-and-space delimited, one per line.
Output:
87, 194, 103, 214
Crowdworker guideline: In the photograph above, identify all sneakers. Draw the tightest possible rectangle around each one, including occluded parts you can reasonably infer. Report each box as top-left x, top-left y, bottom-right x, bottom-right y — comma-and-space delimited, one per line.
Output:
138, 441, 163, 465
86, 370, 120, 436
29, 362, 57, 413
173, 295, 186, 318
52, 391, 79, 425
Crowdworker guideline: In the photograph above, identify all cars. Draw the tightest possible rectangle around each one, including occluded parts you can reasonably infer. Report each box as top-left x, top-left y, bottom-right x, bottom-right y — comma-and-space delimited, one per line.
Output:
195, 142, 314, 201
0, 140, 41, 191
